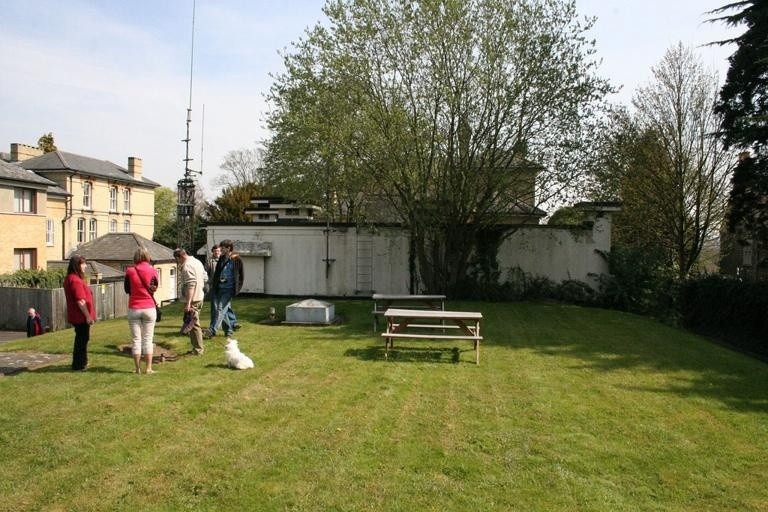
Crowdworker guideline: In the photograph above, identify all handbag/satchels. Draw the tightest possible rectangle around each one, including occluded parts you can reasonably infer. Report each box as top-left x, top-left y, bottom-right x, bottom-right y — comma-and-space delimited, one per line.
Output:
156, 304, 162, 322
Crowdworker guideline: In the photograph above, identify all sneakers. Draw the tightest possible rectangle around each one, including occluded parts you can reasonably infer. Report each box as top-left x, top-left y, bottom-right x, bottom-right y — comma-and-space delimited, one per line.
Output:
225, 324, 241, 336
72, 361, 88, 370
180, 309, 197, 334
136, 368, 158, 375
201, 328, 215, 339
184, 351, 200, 356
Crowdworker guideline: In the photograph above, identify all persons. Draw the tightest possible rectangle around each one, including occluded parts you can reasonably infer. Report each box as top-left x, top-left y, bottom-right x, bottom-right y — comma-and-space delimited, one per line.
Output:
63, 253, 98, 373
25, 308, 46, 340
173, 247, 210, 359
200, 239, 245, 339
123, 246, 160, 375
202, 244, 242, 332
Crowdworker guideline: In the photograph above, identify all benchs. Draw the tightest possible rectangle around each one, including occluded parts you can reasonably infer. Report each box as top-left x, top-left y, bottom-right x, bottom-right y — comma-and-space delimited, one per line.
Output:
371, 293, 484, 366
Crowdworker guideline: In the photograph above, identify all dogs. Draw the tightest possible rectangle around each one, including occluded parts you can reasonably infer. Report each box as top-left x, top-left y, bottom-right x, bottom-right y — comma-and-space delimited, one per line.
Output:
223, 336, 254, 371
